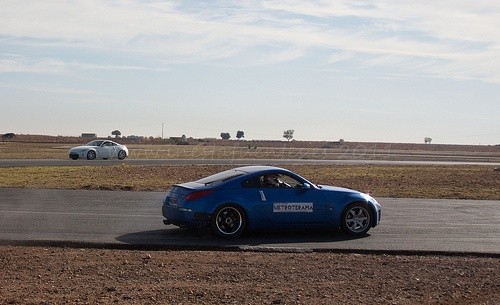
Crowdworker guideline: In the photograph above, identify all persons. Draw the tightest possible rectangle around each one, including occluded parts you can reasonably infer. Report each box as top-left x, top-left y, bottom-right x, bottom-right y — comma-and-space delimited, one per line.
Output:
263, 173, 291, 188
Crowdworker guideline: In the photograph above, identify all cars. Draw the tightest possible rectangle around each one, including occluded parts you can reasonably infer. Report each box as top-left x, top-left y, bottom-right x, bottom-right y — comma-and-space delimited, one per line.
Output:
68, 140, 129, 160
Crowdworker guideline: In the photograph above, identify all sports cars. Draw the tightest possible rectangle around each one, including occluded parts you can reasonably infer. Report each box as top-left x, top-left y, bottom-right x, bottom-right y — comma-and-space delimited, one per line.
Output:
162, 166, 381, 241
